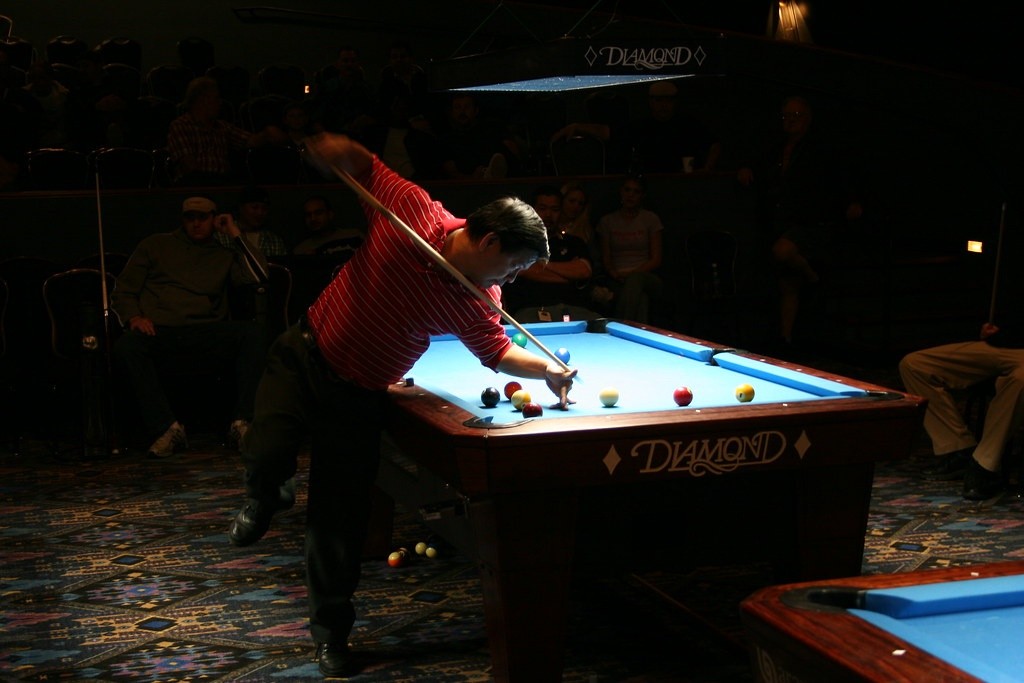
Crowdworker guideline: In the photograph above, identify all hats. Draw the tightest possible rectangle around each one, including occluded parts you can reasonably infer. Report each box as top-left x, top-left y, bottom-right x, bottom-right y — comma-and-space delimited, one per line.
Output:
180, 196, 216, 216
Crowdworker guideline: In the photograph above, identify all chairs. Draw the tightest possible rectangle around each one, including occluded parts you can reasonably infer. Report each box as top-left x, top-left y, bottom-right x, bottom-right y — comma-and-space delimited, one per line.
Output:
381, 63, 425, 79
263, 262, 293, 343
94, 147, 156, 190
313, 65, 336, 90
142, 63, 196, 98
549, 135, 606, 177
91, 37, 142, 71
0, 14, 15, 43
175, 36, 215, 76
0, 35, 34, 73
122, 96, 175, 148
41, 269, 128, 460
683, 229, 742, 347
24, 147, 89, 192
245, 142, 303, 185
257, 62, 307, 101
44, 35, 90, 64
206, 61, 251, 104
247, 93, 299, 133
92, 62, 151, 111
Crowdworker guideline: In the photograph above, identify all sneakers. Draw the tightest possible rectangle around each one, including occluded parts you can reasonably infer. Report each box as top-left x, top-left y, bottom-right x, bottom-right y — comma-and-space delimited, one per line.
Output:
149, 422, 188, 457
229, 420, 249, 442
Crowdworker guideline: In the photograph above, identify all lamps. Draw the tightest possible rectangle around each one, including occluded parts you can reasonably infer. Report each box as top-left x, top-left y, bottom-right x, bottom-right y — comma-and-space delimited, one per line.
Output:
427, 39, 728, 94
765, 0, 816, 47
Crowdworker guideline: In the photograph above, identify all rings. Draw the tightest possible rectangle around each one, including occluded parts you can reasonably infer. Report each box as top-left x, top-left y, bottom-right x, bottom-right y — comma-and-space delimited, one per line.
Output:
147, 321, 154, 327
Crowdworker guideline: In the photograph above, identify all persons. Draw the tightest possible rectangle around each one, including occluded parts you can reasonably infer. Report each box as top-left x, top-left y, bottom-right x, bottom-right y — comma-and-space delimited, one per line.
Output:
228, 133, 577, 675
0, 40, 889, 458
898, 283, 1024, 500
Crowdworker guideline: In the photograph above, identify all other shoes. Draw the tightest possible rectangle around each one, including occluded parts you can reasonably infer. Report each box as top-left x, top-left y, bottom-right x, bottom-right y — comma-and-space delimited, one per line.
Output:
963, 470, 983, 499
947, 450, 968, 479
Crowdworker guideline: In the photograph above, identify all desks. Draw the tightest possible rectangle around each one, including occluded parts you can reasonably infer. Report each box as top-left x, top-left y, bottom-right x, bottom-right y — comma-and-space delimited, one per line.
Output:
357, 310, 928, 683
733, 558, 1024, 683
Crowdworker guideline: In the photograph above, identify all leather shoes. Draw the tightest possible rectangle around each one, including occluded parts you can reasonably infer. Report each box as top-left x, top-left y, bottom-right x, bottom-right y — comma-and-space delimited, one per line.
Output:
228, 502, 270, 545
314, 639, 351, 676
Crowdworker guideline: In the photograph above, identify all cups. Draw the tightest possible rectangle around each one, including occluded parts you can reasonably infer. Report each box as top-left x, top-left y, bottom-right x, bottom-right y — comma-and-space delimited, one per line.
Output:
682, 157, 695, 174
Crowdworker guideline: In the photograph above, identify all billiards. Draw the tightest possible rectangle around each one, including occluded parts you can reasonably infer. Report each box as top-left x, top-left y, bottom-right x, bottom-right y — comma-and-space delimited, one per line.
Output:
425, 547, 437, 558
511, 390, 532, 411
387, 551, 401, 568
522, 401, 543, 419
673, 385, 694, 407
481, 386, 500, 407
599, 385, 619, 407
395, 546, 411, 564
554, 347, 571, 365
415, 542, 428, 555
735, 383, 755, 402
511, 332, 528, 349
504, 381, 522, 400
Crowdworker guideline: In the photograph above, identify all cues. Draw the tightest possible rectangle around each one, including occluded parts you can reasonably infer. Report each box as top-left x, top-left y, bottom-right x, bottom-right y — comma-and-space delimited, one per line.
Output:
325, 162, 585, 386
988, 201, 1007, 327
95, 172, 121, 457
